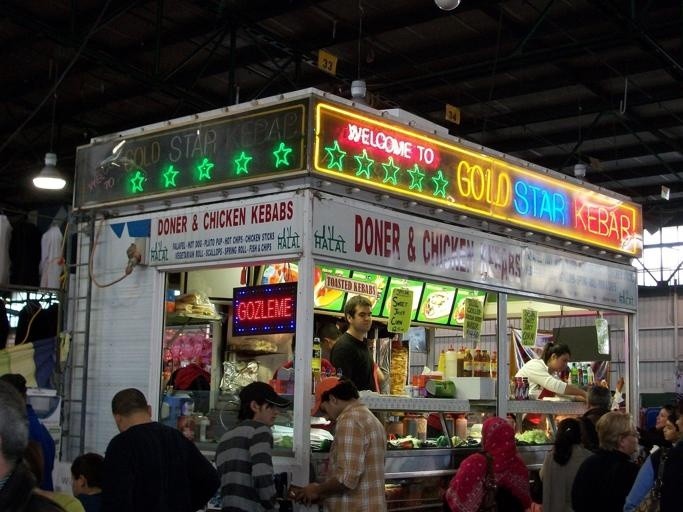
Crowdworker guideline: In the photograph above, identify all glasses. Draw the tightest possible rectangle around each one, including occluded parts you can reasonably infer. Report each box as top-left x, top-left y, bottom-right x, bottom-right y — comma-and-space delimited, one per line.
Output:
616, 429, 642, 440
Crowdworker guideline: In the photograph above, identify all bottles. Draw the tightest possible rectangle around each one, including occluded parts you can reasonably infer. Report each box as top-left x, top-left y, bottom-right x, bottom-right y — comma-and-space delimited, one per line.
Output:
443, 415, 454, 437
509, 376, 529, 400
455, 415, 467, 440
437, 344, 498, 377
387, 412, 428, 441
569, 362, 594, 386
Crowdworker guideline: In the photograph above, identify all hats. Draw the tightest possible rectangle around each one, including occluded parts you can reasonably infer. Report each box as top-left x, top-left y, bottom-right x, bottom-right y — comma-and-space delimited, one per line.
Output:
309, 376, 340, 418
238, 381, 293, 409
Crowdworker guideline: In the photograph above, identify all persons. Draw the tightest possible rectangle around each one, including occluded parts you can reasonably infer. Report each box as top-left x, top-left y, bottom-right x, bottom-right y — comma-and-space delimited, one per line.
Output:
445, 416, 532, 512
328, 294, 377, 392
294, 375, 388, 511
532, 385, 682, 512
98, 387, 220, 511
210, 381, 293, 512
0, 373, 55, 493
69, 452, 106, 511
271, 331, 336, 379
0, 377, 65, 511
24, 439, 84, 511
509, 340, 586, 431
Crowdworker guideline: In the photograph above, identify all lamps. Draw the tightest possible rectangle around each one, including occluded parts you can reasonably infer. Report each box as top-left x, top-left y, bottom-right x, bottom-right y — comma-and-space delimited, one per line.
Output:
30, 85, 69, 194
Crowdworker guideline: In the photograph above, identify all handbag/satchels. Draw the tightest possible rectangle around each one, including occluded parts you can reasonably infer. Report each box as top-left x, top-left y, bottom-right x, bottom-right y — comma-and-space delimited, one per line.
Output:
634, 446, 669, 512
477, 450, 521, 512
